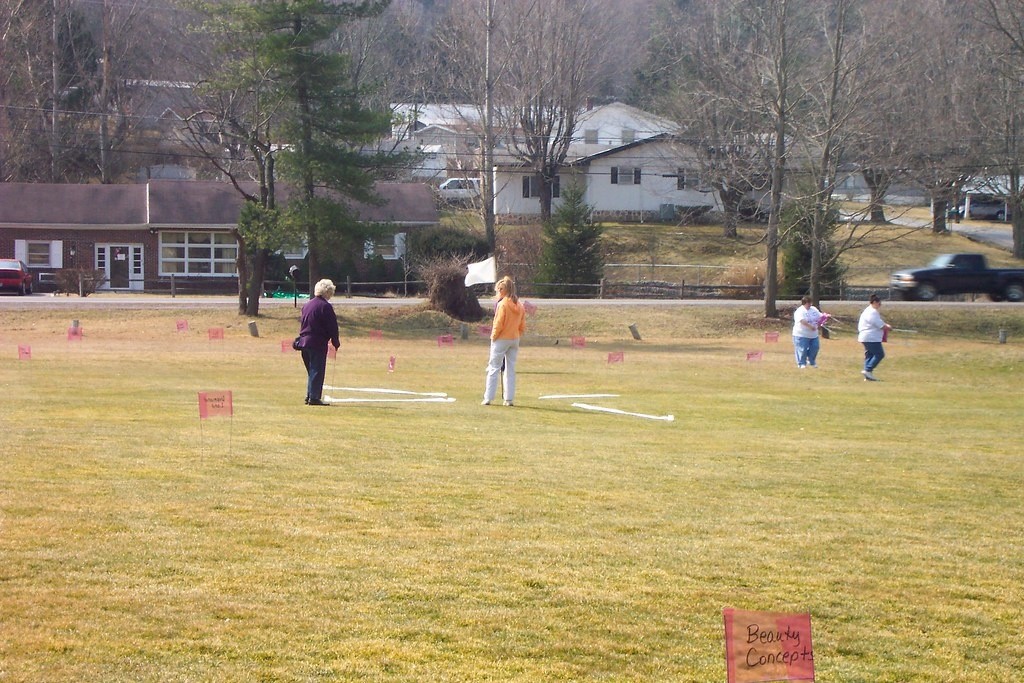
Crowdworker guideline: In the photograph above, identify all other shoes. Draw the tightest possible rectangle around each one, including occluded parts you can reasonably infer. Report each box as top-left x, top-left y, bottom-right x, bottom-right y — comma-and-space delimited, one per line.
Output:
861, 370, 877, 381
808, 360, 818, 367
481, 399, 490, 405
503, 399, 513, 406
305, 396, 330, 406
798, 364, 805, 368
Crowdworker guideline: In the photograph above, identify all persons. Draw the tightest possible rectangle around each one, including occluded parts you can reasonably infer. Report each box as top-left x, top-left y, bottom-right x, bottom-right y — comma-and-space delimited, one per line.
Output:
479, 276, 526, 406
858, 294, 893, 380
300, 279, 340, 405
793, 295, 832, 368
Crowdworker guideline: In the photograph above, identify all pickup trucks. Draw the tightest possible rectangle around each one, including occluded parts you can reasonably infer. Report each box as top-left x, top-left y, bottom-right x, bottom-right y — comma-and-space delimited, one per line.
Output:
890, 251, 1024, 302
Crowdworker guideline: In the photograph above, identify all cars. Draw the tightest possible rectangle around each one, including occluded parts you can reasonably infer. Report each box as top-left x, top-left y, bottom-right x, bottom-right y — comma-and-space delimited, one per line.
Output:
0, 259, 34, 296
950, 196, 1022, 223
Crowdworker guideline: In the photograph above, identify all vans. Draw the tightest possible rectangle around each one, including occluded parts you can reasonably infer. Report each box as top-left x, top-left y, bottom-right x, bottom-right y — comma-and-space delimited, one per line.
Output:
437, 177, 484, 209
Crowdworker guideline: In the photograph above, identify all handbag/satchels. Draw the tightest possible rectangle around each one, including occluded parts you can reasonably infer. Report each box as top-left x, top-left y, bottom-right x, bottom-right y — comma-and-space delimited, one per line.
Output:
292, 337, 301, 350
